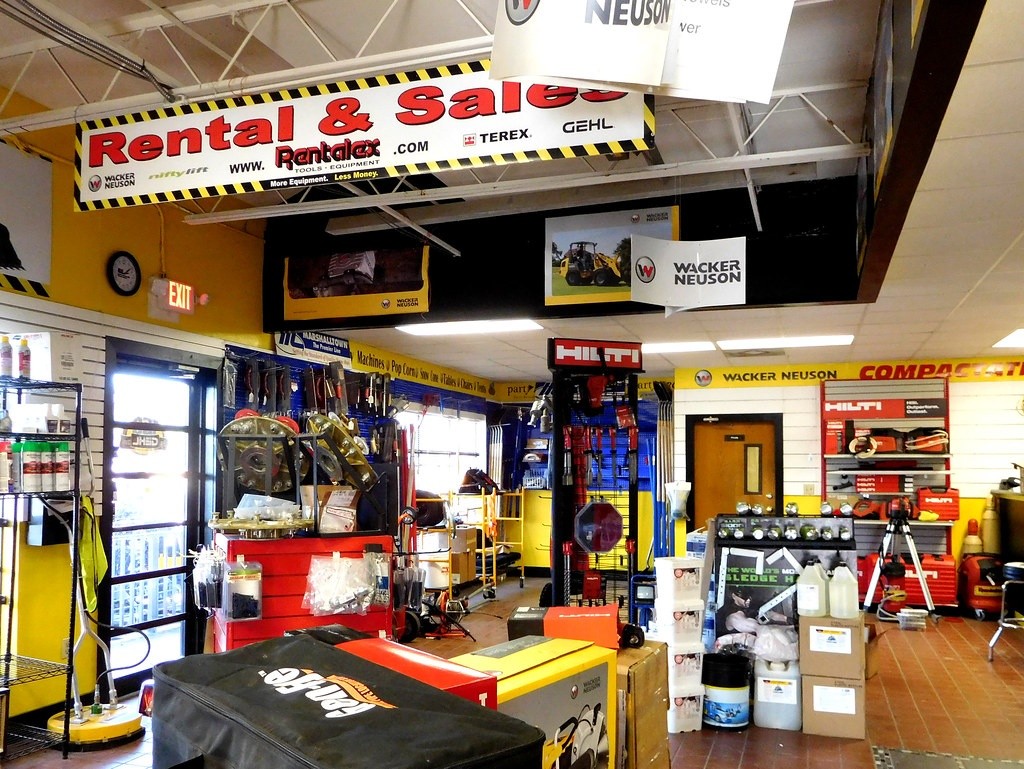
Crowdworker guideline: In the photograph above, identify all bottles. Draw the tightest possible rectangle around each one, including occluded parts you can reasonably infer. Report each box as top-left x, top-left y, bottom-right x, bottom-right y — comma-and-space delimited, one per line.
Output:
813, 558, 830, 615
0, 410, 12, 432
829, 562, 859, 619
19, 339, 31, 379
0, 337, 13, 377
797, 560, 825, 616
0, 442, 70, 493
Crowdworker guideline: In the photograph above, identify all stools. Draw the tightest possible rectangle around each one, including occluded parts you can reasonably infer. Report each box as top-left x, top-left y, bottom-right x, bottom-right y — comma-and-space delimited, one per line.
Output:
988, 562, 1024, 661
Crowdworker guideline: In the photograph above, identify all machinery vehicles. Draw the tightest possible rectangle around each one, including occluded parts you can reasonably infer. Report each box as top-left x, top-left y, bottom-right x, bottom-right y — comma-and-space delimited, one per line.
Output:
559, 240, 624, 286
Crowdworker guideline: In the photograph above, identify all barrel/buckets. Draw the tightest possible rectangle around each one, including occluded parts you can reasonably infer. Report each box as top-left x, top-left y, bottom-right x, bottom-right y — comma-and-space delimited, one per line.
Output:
703, 653, 751, 732
754, 661, 802, 731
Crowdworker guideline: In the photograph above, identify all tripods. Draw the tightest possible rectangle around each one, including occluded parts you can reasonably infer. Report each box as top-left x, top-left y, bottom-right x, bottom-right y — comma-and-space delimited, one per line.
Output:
862, 509, 938, 623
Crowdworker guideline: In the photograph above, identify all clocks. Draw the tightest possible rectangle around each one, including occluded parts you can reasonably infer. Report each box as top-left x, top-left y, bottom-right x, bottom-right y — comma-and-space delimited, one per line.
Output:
108, 251, 141, 296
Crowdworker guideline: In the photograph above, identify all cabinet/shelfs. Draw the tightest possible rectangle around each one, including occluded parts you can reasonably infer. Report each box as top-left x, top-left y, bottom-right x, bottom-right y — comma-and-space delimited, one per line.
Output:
455, 487, 526, 587
823, 453, 954, 526
0, 377, 84, 764
411, 488, 485, 604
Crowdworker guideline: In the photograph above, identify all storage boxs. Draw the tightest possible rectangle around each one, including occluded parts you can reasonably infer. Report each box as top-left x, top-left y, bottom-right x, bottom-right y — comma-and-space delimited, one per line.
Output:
800, 612, 866, 680
801, 674, 865, 740
286, 486, 704, 769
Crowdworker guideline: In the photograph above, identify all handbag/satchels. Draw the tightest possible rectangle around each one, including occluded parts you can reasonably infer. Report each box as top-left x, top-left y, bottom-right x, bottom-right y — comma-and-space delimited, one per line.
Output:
153, 632, 544, 769
